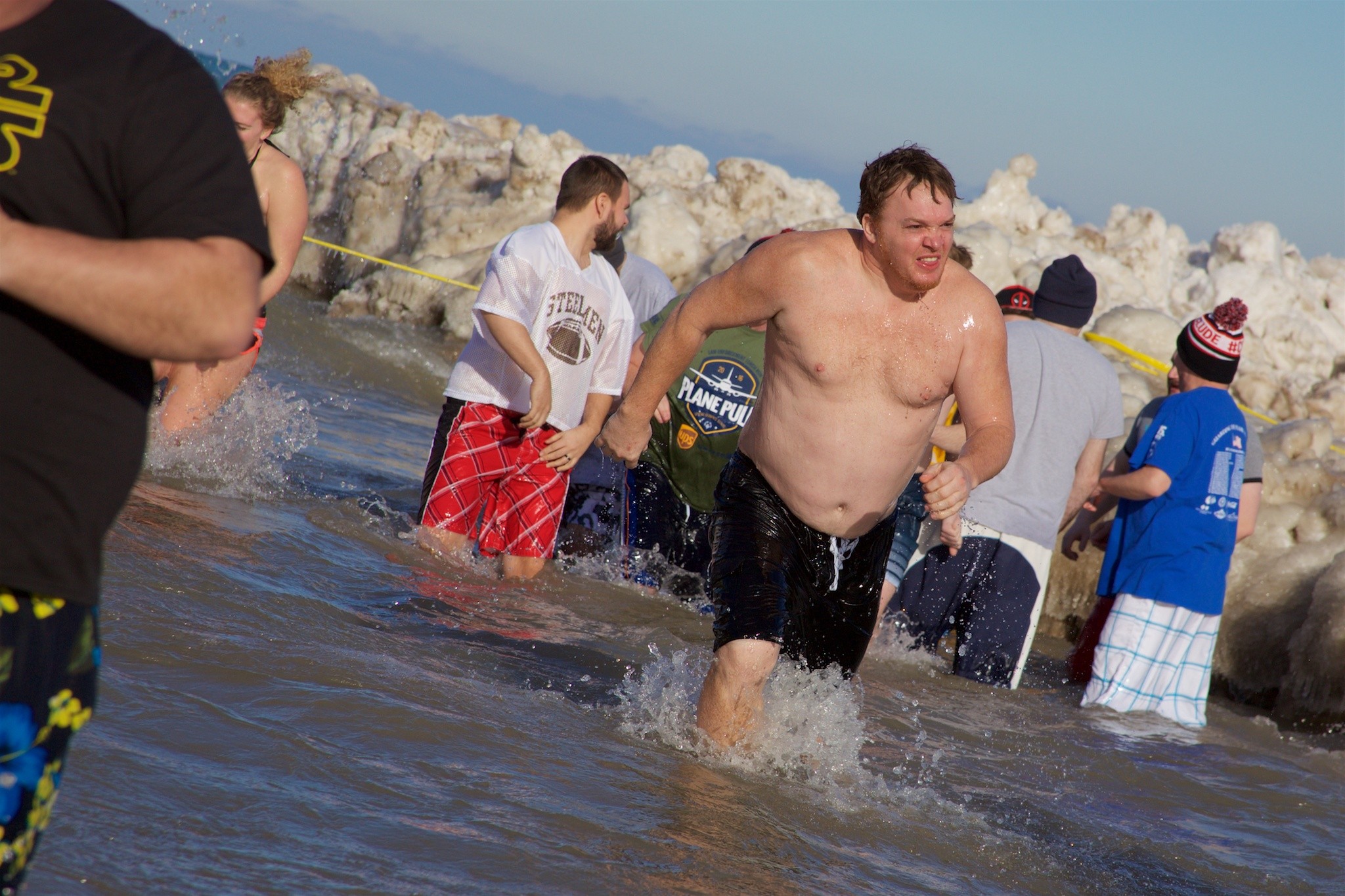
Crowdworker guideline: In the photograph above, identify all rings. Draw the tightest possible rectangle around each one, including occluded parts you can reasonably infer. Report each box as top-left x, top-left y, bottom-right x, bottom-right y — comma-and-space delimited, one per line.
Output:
564, 454, 570, 460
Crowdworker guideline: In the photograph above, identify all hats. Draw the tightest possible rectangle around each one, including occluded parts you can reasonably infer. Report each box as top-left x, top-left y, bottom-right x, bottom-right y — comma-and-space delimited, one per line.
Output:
995, 285, 1035, 316
1176, 298, 1249, 384
1032, 255, 1098, 327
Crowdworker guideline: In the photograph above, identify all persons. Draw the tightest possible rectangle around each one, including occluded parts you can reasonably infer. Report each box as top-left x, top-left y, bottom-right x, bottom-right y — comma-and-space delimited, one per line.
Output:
596, 140, 1016, 756
1061, 348, 1263, 685
0, 0, 276, 896
620, 228, 799, 604
875, 254, 1125, 691
416, 155, 634, 579
905, 286, 1033, 660
150, 44, 337, 447
1080, 295, 1249, 729
553, 231, 676, 571
873, 240, 976, 636
